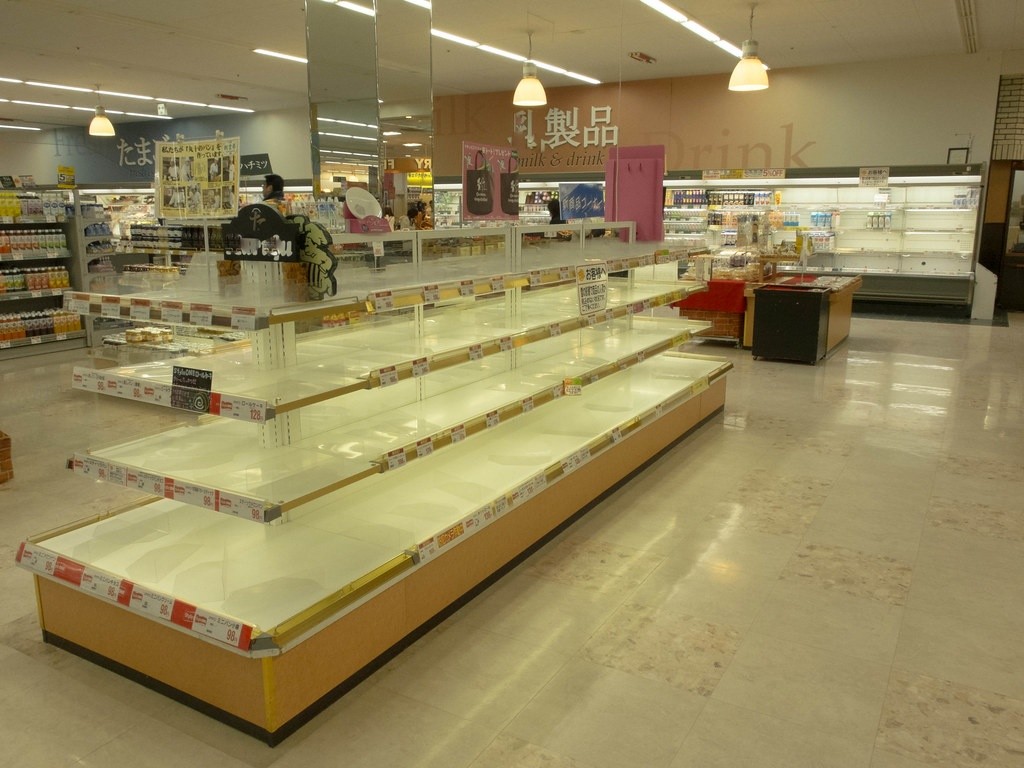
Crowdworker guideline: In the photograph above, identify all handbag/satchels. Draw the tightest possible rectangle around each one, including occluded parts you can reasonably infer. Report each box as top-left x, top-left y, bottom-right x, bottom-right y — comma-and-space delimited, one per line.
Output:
467, 150, 493, 215
501, 156, 519, 215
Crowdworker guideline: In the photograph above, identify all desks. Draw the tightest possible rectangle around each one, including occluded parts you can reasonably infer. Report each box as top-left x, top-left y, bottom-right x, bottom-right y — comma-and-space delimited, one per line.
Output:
670, 273, 773, 349
743, 272, 864, 356
754, 284, 832, 367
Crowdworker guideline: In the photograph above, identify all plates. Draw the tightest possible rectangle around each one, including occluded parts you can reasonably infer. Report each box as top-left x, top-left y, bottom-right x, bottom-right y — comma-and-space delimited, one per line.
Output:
345, 187, 382, 219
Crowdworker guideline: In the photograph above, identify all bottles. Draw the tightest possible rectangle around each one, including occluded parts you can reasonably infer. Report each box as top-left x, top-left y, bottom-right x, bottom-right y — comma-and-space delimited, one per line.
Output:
0, 229, 67, 254
0, 266, 69, 290
0, 191, 66, 217
0, 308, 81, 341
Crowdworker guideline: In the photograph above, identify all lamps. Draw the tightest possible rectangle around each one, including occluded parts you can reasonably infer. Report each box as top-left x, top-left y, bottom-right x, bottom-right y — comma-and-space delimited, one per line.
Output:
728, 3, 769, 91
88, 84, 115, 137
512, 31, 547, 106
638, 0, 773, 71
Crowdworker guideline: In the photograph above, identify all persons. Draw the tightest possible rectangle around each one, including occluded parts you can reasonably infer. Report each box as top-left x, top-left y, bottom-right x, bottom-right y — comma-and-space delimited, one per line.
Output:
213, 189, 220, 208
547, 201, 566, 224
188, 185, 200, 208
227, 186, 235, 206
167, 157, 191, 182
224, 156, 234, 179
262, 175, 285, 208
407, 209, 418, 219
209, 158, 219, 179
169, 185, 185, 207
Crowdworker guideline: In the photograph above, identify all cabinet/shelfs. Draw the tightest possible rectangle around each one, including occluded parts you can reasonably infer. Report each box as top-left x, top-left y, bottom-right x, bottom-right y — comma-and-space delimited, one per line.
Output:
0, 164, 987, 750
